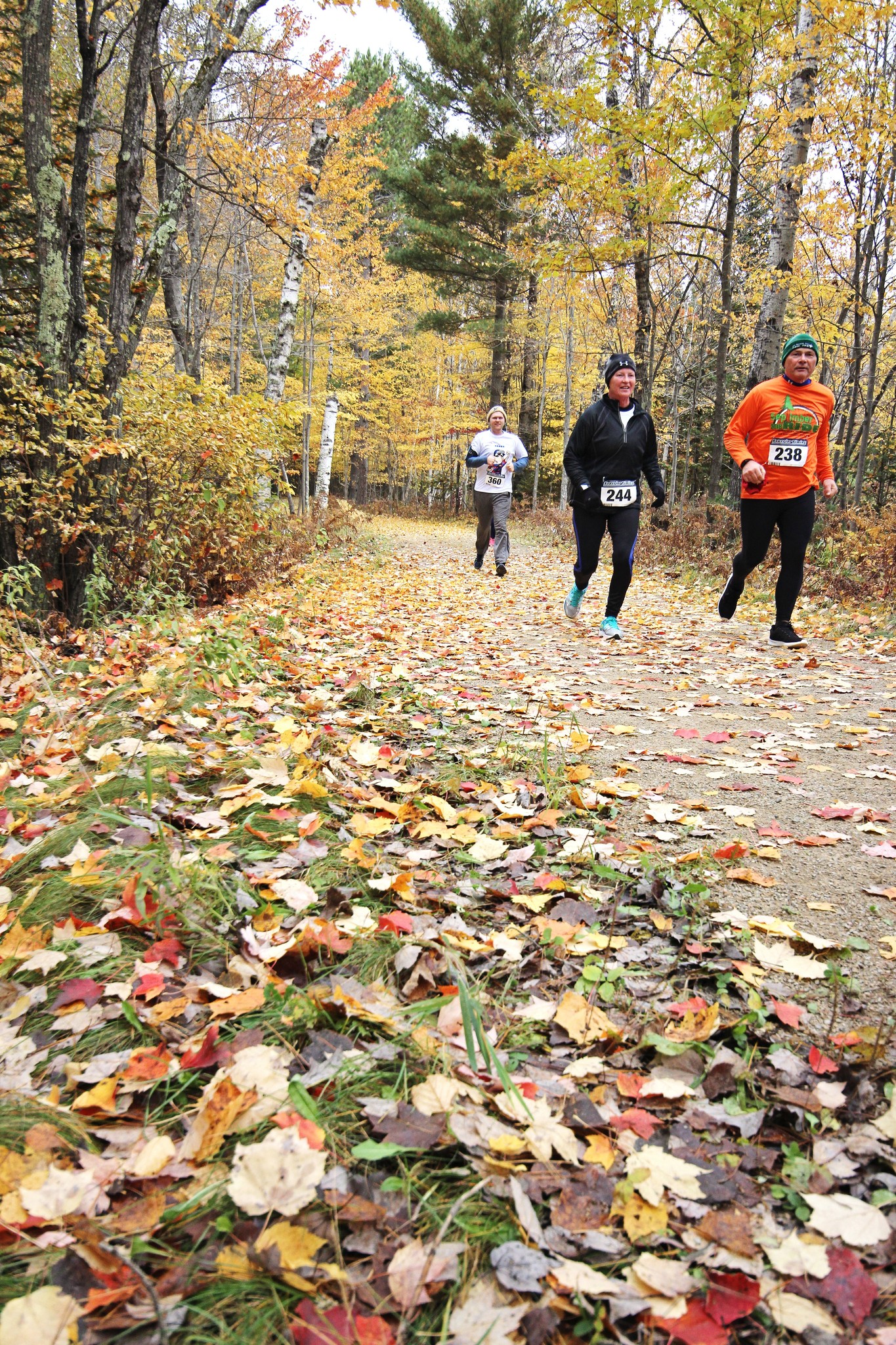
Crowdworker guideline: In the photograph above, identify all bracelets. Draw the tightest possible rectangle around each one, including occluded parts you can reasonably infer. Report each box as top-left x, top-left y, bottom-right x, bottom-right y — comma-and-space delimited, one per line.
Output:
741, 459, 751, 468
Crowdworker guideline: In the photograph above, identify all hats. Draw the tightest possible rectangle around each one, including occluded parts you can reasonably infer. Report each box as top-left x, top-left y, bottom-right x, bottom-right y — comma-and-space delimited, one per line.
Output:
605, 353, 636, 389
487, 406, 507, 424
782, 334, 819, 369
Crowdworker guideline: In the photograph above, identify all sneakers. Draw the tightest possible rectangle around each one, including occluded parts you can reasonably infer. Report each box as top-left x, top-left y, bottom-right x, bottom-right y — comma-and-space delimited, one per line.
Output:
564, 582, 589, 619
489, 538, 495, 546
474, 554, 484, 570
768, 622, 808, 649
598, 617, 623, 640
718, 572, 745, 621
495, 564, 507, 577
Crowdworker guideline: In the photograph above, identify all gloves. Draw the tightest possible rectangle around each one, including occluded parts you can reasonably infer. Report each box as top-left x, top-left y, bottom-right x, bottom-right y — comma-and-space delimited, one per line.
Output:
582, 487, 600, 510
650, 486, 666, 509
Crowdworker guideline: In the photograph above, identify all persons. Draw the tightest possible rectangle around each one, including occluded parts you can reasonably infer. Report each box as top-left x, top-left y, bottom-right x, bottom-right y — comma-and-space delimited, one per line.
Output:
718, 334, 838, 645
562, 353, 665, 641
466, 406, 528, 577
489, 517, 496, 547
